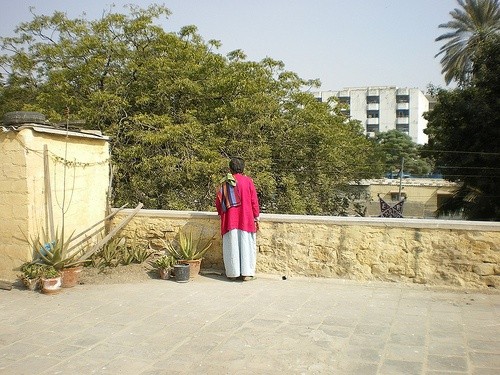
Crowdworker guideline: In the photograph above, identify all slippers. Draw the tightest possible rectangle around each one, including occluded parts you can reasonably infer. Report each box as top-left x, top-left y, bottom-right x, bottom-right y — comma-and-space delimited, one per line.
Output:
231, 276, 241, 280
243, 276, 257, 280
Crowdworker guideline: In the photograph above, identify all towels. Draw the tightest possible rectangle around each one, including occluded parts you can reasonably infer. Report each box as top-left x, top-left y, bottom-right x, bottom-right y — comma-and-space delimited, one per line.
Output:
216, 181, 242, 214
217, 172, 238, 187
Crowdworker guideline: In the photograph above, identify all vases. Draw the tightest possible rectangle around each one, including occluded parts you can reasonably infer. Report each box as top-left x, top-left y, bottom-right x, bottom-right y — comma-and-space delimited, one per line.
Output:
173, 263, 190, 283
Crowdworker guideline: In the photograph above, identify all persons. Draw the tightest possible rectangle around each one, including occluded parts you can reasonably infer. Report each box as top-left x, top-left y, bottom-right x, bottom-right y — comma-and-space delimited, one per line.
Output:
215, 156, 260, 281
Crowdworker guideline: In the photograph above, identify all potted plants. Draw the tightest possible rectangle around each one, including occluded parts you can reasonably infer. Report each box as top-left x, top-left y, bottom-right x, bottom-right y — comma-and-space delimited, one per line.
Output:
161, 226, 218, 278
17, 262, 42, 291
155, 256, 172, 280
41, 266, 61, 294
19, 225, 92, 289
